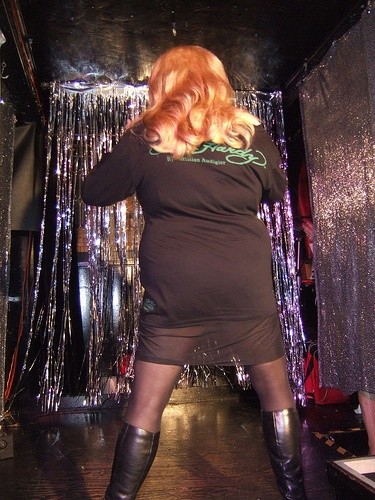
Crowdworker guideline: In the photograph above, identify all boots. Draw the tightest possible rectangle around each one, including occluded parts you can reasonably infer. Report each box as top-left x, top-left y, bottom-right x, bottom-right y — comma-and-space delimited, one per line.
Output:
104, 423, 160, 500
262, 409, 308, 500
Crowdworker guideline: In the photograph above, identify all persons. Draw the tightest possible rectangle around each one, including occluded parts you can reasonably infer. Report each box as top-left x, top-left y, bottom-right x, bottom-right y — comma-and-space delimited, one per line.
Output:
80, 44, 310, 500
302, 219, 375, 458
